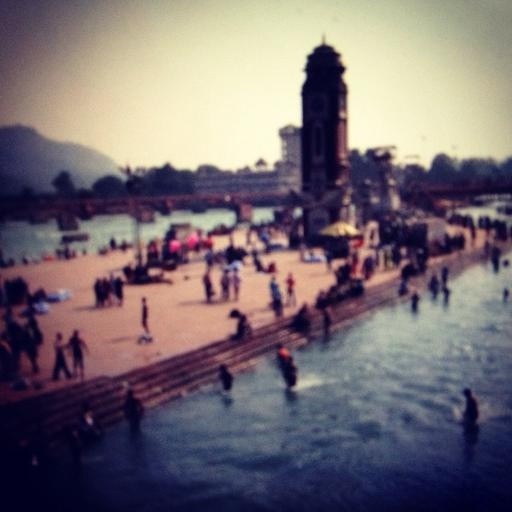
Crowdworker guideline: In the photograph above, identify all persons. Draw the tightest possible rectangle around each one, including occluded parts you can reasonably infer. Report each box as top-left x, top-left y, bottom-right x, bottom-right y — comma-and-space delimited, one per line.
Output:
1, 207, 511, 448
